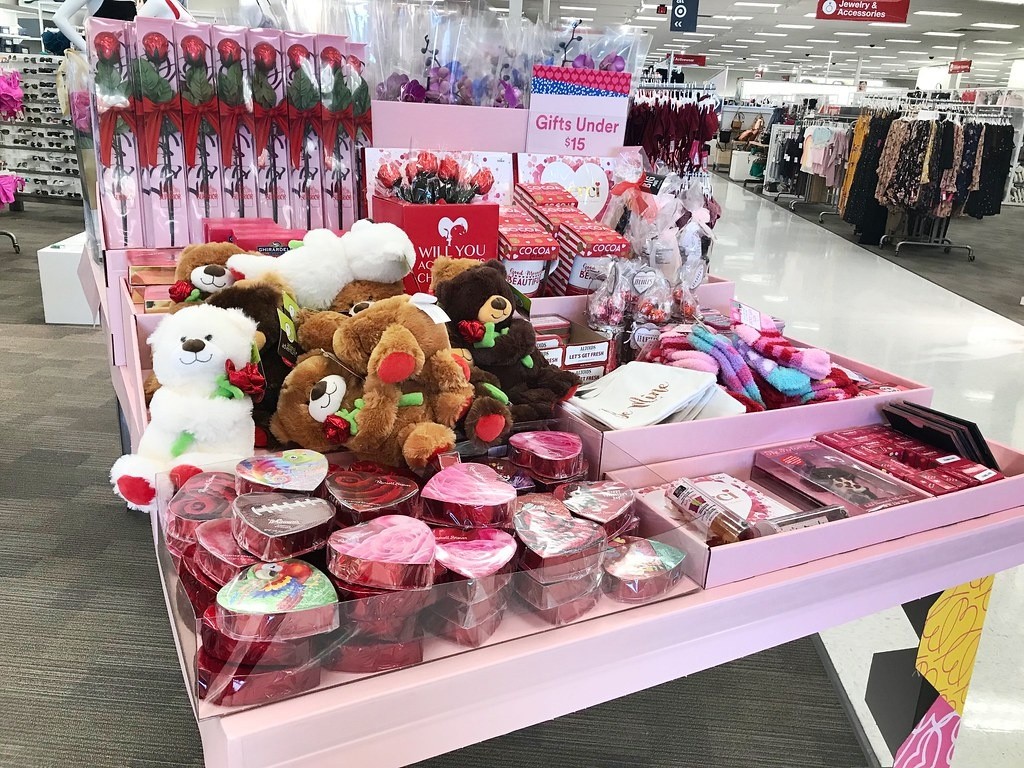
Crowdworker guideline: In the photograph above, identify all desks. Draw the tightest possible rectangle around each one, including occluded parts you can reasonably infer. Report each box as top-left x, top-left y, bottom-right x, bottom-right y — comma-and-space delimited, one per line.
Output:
713, 148, 731, 171
115, 394, 1024, 768
728, 150, 760, 183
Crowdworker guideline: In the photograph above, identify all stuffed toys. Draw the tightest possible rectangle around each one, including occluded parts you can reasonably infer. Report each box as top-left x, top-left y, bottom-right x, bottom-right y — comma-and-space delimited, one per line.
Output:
143, 219, 583, 477
109, 303, 257, 512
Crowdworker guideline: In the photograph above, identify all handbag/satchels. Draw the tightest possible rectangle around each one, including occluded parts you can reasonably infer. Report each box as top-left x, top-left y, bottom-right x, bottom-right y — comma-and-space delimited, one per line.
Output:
719, 110, 767, 152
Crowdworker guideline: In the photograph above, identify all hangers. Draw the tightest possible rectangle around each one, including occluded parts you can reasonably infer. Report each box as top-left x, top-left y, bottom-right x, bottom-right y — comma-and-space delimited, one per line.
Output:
634, 79, 720, 115
777, 93, 1012, 142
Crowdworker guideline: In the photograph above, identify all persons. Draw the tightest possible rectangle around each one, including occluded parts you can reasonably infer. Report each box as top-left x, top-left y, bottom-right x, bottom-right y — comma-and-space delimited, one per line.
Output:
240, 0, 283, 29
51, 0, 195, 50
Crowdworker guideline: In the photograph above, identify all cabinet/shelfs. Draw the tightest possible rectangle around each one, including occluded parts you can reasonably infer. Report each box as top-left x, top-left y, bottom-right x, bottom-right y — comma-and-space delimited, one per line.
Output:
0, 0, 87, 52
909, 88, 1024, 206
0, 52, 84, 212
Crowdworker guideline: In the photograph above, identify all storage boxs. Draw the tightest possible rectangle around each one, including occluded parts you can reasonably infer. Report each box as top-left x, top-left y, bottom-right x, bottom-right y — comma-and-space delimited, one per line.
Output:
527, 205, 594, 241
620, 146, 654, 173
528, 273, 736, 326
370, 194, 500, 294
514, 153, 620, 224
557, 222, 630, 257
370, 98, 530, 153
553, 336, 935, 481
496, 204, 535, 226
604, 423, 1024, 590
515, 313, 611, 387
84, 16, 374, 433
512, 183, 579, 212
498, 224, 560, 261
530, 315, 572, 334
0, 25, 30, 54
360, 147, 513, 220
